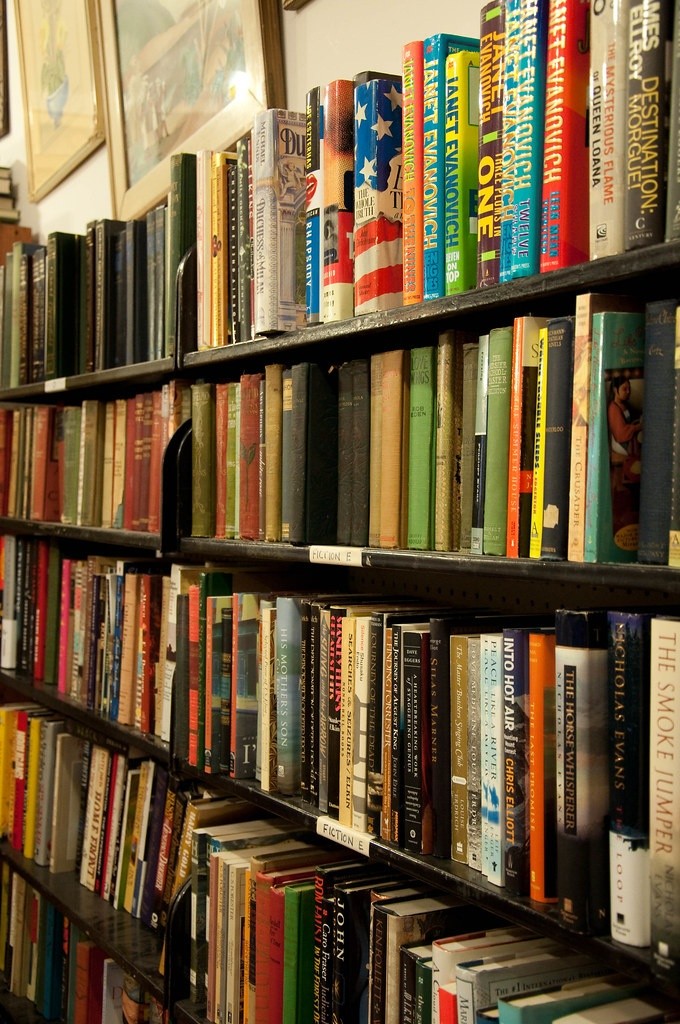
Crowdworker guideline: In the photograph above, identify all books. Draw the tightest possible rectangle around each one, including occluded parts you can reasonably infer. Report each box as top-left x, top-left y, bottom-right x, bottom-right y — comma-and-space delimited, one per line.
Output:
0, 1, 680, 1024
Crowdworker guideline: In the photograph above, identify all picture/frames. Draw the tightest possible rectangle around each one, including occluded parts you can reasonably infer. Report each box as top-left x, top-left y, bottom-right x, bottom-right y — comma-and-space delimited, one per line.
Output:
86, 0, 288, 222
11, 1, 107, 203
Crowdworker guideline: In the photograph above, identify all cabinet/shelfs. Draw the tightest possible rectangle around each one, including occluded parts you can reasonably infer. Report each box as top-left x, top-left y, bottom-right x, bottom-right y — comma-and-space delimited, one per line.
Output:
0, 0, 680, 1023
